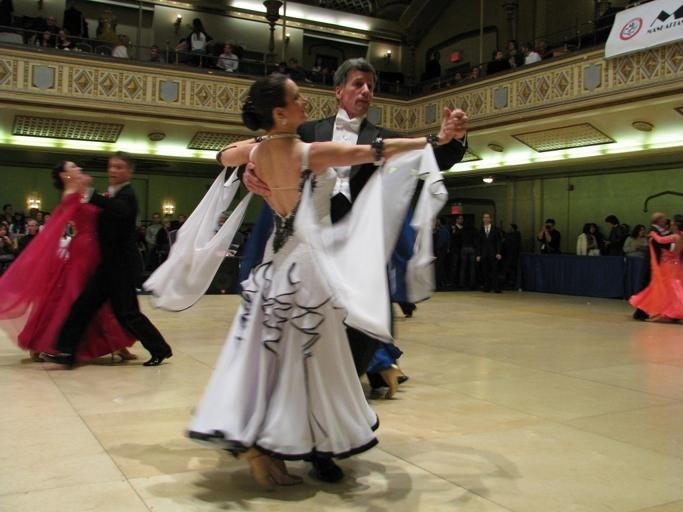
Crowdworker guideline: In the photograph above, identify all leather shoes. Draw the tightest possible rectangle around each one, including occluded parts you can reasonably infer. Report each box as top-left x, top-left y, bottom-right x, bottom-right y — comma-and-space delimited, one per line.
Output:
39, 352, 74, 364
143, 343, 173, 366
311, 458, 344, 483
371, 375, 408, 389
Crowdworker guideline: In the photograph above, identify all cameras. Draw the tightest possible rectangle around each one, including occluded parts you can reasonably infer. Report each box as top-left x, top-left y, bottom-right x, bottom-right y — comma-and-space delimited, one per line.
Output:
0, 237, 4, 241
545, 225, 552, 230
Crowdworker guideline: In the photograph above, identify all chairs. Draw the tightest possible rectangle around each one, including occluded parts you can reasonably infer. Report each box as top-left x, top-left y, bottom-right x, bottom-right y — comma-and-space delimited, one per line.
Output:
413, 6, 627, 101
59, 29, 412, 100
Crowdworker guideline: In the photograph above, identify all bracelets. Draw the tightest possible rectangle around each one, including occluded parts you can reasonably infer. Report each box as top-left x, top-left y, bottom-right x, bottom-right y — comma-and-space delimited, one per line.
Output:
423, 133, 440, 149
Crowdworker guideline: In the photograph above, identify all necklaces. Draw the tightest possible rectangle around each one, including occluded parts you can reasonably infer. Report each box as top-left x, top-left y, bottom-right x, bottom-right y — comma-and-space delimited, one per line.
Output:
254, 132, 302, 142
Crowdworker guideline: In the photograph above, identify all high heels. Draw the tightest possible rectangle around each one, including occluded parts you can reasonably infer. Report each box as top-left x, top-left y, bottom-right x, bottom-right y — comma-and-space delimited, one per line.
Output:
379, 363, 407, 400
246, 445, 303, 491
111, 346, 137, 360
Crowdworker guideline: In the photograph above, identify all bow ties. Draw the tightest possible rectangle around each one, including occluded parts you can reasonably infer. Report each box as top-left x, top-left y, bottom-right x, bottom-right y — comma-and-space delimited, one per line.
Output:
335, 115, 361, 134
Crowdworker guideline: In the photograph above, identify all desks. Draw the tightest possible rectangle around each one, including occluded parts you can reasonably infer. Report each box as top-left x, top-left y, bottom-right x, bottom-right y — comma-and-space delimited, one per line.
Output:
627, 247, 653, 295
516, 242, 629, 304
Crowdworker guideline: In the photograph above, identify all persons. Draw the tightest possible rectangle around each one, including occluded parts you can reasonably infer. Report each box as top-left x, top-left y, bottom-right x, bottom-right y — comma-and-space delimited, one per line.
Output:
22, 15, 82, 52
433, 214, 467, 291
295, 58, 466, 482
576, 211, 683, 324
472, 212, 561, 293
136, 208, 244, 294
0, 158, 140, 369
267, 57, 344, 87
149, 18, 239, 72
1, 204, 51, 275
142, 74, 459, 489
410, 40, 553, 96
41, 145, 174, 369
112, 34, 130, 58
